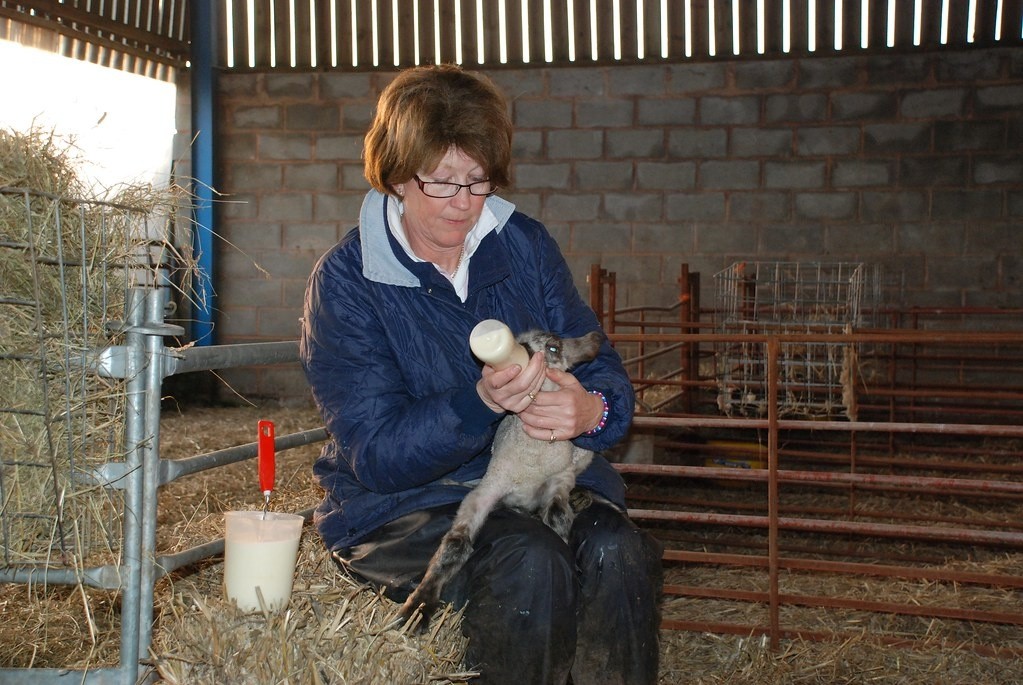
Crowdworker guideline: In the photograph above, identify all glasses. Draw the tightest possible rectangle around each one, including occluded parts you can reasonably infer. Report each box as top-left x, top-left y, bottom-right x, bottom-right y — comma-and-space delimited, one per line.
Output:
414, 175, 498, 198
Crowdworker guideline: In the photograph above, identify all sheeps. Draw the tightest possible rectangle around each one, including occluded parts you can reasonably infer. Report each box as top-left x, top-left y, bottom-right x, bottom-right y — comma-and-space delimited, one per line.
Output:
398, 327, 604, 631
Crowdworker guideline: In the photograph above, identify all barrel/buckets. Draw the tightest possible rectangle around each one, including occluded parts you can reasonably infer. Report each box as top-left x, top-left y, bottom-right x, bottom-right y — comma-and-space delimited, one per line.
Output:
222, 510, 305, 615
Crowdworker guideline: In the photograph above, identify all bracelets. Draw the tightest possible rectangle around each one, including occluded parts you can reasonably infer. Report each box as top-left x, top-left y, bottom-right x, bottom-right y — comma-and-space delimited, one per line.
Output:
584, 391, 609, 435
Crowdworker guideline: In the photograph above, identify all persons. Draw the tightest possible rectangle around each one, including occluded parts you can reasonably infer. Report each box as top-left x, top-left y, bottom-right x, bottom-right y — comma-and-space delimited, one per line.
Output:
299, 66, 662, 685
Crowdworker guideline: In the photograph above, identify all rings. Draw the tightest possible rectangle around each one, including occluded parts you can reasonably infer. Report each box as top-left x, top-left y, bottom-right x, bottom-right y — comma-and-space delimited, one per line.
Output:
528, 392, 537, 402
548, 429, 556, 444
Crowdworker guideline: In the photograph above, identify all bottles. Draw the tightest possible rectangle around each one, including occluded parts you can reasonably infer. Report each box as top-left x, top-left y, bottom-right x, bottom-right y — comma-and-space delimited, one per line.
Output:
469, 319, 531, 379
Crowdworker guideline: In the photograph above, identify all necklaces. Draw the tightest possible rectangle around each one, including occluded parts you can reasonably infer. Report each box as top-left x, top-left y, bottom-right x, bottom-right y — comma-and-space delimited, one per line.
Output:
400, 214, 464, 279
